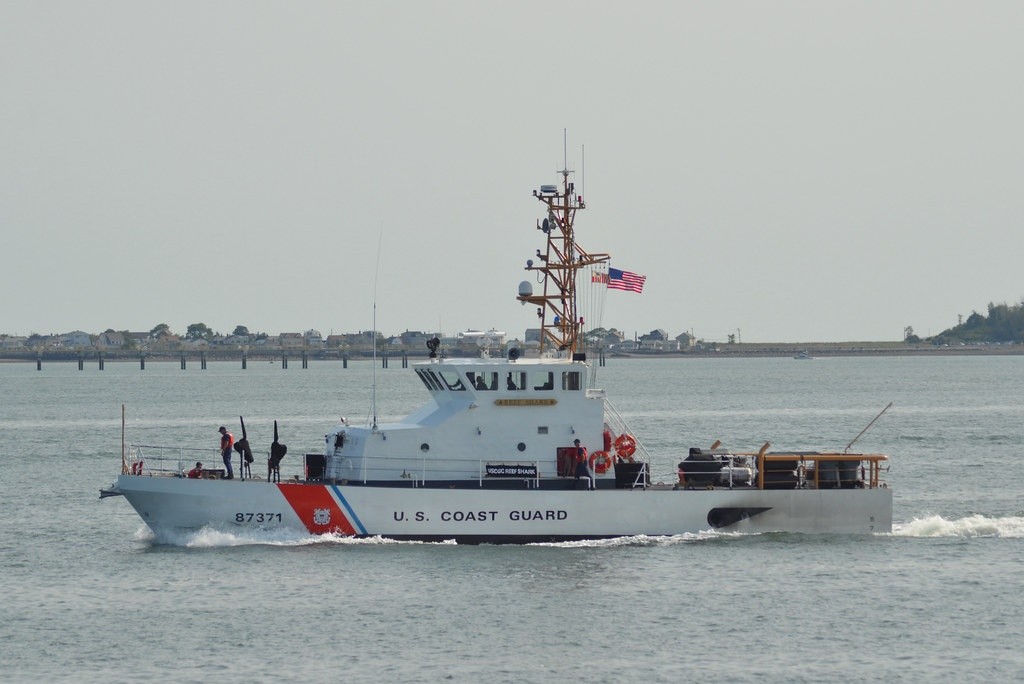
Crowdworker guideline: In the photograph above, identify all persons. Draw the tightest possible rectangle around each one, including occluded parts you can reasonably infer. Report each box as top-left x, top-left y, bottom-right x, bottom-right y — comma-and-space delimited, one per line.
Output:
449, 375, 519, 391
188, 462, 204, 477
218, 427, 235, 480
573, 439, 594, 489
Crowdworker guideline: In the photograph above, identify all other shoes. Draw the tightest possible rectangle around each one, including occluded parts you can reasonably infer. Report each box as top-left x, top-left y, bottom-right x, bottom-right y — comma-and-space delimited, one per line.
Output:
225, 474, 234, 479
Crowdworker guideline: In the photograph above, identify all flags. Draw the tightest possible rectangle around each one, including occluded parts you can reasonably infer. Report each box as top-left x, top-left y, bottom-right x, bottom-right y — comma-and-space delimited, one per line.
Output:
591, 270, 609, 283
607, 268, 646, 293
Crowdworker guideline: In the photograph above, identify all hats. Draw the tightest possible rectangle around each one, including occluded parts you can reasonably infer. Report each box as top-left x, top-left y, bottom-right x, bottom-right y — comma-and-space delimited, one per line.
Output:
573, 439, 581, 442
217, 427, 226, 433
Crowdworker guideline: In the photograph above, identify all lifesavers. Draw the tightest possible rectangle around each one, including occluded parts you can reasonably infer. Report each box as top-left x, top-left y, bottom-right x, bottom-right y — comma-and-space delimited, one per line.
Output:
589, 451, 611, 474
615, 435, 636, 458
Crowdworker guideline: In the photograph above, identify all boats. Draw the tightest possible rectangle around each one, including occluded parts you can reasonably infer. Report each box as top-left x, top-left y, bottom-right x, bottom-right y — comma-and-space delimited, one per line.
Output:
114, 124, 892, 546
